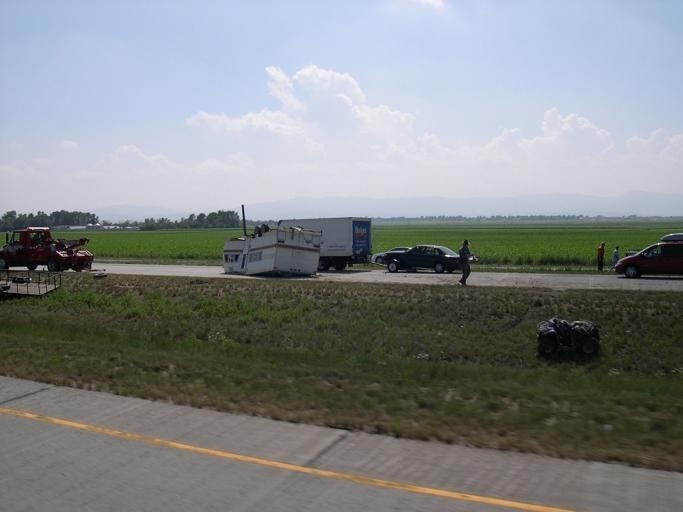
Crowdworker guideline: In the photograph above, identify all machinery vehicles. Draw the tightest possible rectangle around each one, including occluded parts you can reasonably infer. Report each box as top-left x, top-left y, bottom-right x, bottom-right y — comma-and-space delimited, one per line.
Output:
0, 226, 94, 273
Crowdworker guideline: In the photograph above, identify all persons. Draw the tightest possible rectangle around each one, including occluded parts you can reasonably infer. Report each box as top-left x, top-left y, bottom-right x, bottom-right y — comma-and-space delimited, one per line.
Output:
458, 238, 476, 287
597, 241, 606, 273
607, 245, 621, 273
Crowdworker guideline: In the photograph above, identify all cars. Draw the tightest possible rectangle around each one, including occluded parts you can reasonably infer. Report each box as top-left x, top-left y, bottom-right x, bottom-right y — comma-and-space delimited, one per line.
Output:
388, 244, 478, 274
613, 232, 683, 279
371, 247, 413, 265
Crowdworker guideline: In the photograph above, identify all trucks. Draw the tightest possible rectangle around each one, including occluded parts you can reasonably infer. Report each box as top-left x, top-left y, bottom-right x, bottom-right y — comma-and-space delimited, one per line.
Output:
276, 216, 373, 272
